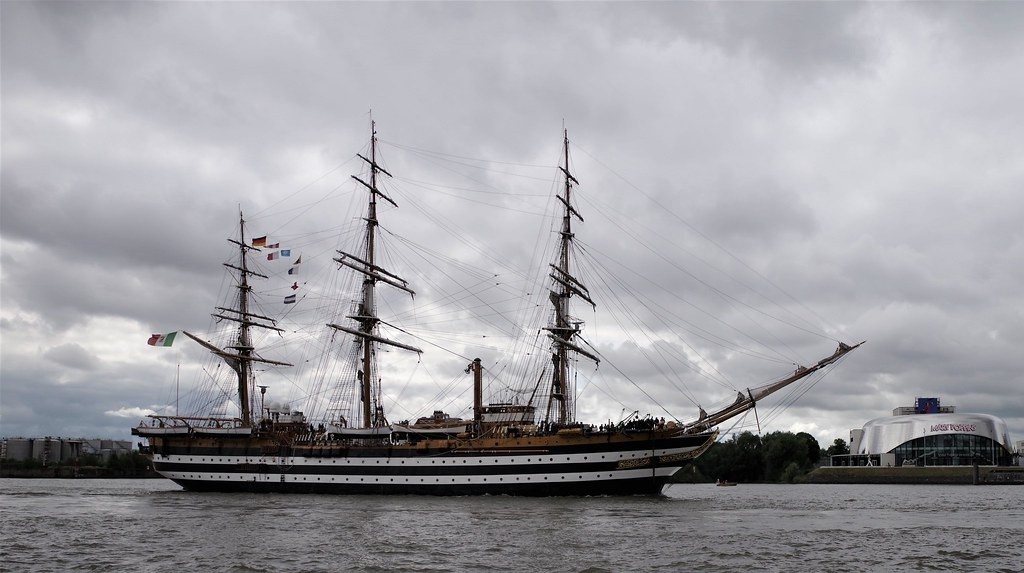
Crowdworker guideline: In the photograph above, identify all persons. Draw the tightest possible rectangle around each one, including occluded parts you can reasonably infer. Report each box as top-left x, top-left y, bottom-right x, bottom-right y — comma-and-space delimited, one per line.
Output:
261, 418, 326, 436
537, 416, 665, 437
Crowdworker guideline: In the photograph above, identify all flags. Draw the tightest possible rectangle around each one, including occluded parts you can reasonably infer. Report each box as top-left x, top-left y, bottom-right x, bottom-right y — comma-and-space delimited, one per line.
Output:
252, 236, 301, 304
148, 332, 177, 346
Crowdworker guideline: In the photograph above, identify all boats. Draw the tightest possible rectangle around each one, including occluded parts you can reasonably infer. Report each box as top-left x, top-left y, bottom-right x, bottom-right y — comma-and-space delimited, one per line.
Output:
716, 482, 738, 486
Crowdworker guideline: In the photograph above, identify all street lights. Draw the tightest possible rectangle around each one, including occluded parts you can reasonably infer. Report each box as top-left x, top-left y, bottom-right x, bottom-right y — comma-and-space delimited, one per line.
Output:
258, 385, 270, 420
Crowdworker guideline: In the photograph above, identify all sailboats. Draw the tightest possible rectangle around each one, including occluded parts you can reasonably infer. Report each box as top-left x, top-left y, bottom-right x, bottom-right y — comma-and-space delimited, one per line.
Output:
130, 109, 867, 501
